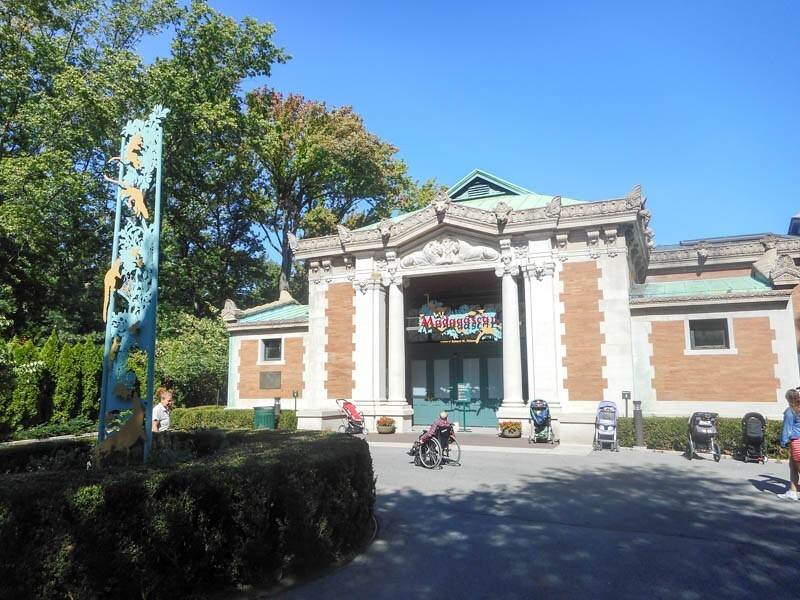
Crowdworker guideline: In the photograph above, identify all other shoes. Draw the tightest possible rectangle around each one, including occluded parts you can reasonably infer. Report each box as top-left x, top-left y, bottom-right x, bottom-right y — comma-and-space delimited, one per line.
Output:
407, 451, 415, 457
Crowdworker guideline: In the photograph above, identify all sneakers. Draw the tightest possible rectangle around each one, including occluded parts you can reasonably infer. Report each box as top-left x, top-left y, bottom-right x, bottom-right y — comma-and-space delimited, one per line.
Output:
777, 491, 798, 501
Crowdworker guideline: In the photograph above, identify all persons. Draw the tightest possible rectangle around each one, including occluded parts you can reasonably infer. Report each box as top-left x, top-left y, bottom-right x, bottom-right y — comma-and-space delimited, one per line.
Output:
777, 387, 800, 500
151, 389, 173, 432
406, 412, 456, 455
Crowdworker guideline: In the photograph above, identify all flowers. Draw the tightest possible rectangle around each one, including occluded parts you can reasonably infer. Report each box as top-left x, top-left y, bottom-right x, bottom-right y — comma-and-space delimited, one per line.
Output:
498, 422, 524, 433
377, 417, 395, 426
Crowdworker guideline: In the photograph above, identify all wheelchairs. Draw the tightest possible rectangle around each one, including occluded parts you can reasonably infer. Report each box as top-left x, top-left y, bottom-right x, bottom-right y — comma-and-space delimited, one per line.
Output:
410, 423, 461, 470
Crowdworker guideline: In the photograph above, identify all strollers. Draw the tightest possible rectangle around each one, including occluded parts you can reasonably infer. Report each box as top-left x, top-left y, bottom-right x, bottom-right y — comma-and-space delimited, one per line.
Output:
529, 400, 554, 444
686, 412, 721, 462
593, 400, 621, 452
741, 412, 768, 464
336, 398, 369, 436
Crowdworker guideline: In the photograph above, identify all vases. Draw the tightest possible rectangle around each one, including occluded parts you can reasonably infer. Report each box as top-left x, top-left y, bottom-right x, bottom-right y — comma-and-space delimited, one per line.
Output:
501, 431, 521, 439
377, 426, 396, 433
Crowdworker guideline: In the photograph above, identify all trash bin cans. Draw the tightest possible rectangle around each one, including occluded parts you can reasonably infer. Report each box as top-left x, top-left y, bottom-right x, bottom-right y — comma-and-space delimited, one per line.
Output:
254, 406, 275, 429
